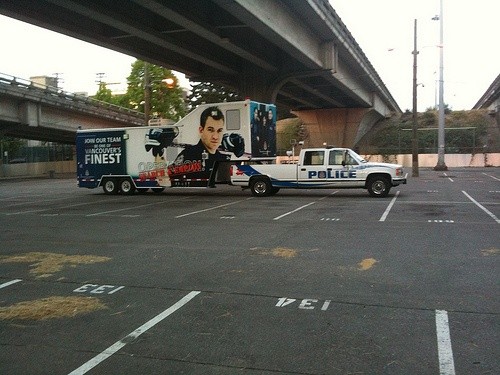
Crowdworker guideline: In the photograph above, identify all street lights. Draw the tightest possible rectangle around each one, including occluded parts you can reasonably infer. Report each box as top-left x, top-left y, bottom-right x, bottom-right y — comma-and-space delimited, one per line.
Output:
387, 44, 443, 176
432, 14, 446, 169
144, 77, 174, 125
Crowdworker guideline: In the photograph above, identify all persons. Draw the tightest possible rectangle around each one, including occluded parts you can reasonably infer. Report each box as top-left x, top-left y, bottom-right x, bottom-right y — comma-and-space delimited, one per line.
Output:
251, 107, 275, 157
166, 106, 245, 168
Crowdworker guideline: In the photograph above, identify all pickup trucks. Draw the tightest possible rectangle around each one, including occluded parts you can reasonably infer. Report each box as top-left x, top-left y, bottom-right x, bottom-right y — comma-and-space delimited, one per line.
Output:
231, 145, 408, 197
